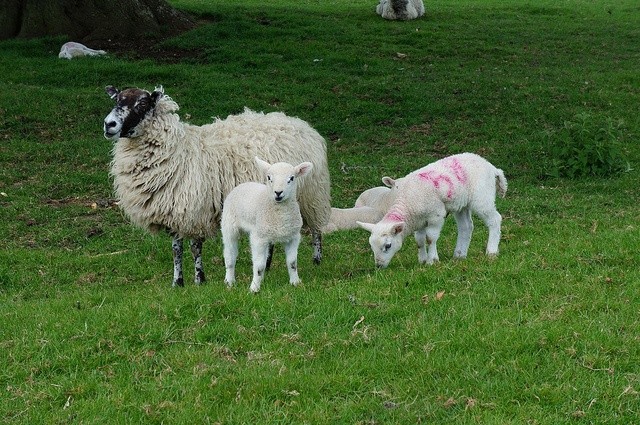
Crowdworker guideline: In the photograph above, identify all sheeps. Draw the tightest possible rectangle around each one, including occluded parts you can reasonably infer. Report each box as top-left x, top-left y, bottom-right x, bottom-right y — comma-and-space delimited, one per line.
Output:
218, 154, 312, 295
355, 176, 404, 210
58, 41, 107, 60
103, 83, 334, 290
320, 206, 384, 234
376, 0, 425, 20
357, 152, 508, 268
